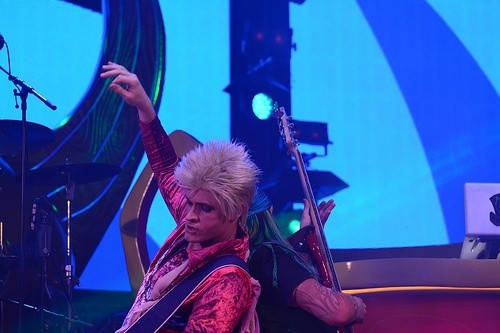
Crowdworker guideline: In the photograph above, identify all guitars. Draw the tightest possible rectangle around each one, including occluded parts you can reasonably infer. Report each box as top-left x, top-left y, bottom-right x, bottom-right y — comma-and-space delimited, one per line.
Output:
271, 100, 355, 333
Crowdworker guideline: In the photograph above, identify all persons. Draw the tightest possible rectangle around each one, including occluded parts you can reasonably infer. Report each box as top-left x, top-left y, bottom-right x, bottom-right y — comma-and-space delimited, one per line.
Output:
237, 184, 367, 333
100, 61, 262, 333
